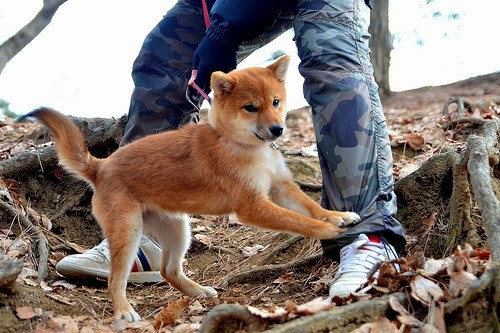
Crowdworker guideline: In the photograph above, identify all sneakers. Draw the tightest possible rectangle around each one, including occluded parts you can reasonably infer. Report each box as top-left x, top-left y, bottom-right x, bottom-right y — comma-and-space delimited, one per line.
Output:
57, 229, 184, 284
329, 234, 402, 301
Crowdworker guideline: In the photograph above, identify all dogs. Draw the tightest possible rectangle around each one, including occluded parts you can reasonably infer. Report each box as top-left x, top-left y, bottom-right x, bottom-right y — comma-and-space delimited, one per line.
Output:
14, 54, 362, 323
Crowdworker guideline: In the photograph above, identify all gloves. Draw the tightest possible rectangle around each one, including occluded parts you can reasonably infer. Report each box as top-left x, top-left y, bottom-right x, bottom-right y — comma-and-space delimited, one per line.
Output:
186, 23, 244, 97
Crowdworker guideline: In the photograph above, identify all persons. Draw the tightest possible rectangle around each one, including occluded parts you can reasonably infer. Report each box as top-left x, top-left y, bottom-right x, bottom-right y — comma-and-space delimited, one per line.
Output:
55, 0, 407, 303
